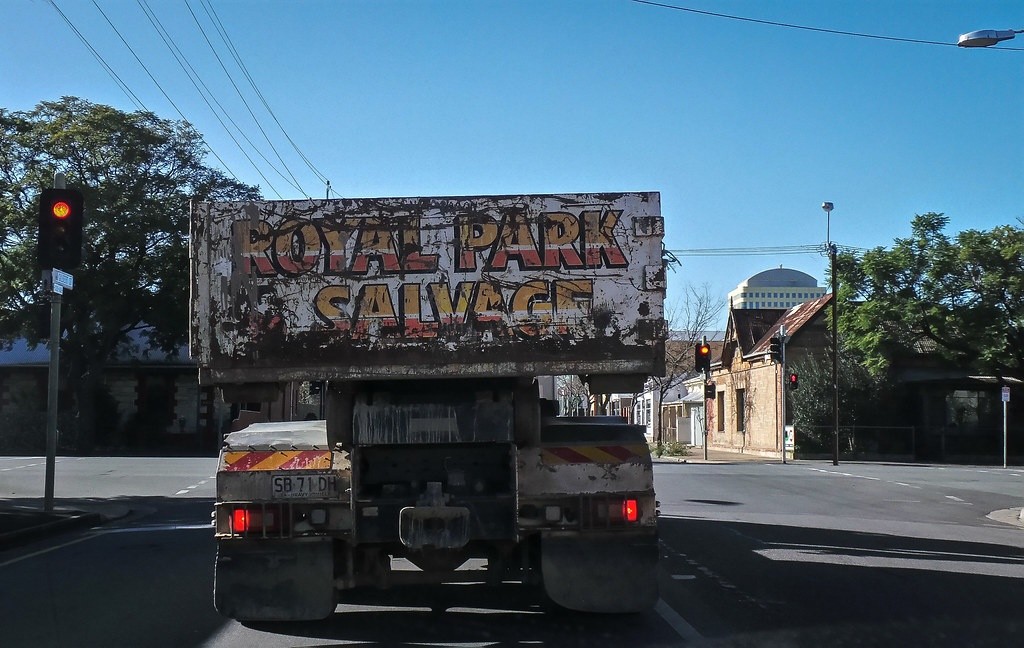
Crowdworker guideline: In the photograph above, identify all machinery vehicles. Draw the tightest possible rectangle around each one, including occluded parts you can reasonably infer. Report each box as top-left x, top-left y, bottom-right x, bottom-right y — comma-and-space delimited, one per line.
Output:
186, 190, 672, 632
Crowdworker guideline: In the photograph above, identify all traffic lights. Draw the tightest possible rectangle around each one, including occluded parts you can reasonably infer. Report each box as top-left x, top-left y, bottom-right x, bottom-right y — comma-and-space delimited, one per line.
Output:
694, 343, 710, 373
38, 188, 83, 269
770, 336, 782, 362
790, 374, 799, 389
